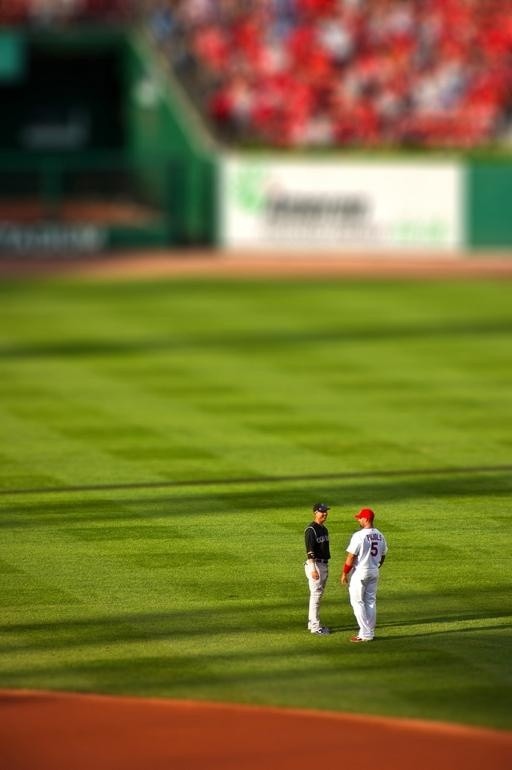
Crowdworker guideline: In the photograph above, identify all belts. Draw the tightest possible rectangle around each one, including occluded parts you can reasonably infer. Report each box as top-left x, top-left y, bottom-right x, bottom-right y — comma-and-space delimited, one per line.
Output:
317, 559, 328, 564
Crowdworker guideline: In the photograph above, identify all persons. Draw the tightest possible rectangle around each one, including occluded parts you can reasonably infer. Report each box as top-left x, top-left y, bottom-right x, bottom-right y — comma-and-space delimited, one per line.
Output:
341, 508, 388, 643
303, 503, 332, 635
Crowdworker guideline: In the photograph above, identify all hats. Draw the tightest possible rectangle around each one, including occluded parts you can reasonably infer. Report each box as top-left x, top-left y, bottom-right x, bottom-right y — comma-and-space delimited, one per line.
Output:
313, 503, 330, 512
354, 508, 374, 519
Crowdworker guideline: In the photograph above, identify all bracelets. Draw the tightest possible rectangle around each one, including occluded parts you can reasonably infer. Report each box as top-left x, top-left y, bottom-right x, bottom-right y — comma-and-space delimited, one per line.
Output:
310, 563, 316, 572
344, 564, 352, 573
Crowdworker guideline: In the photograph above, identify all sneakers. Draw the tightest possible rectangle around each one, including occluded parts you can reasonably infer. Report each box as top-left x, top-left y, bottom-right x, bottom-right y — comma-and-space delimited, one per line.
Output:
351, 636, 365, 642
315, 627, 330, 636
